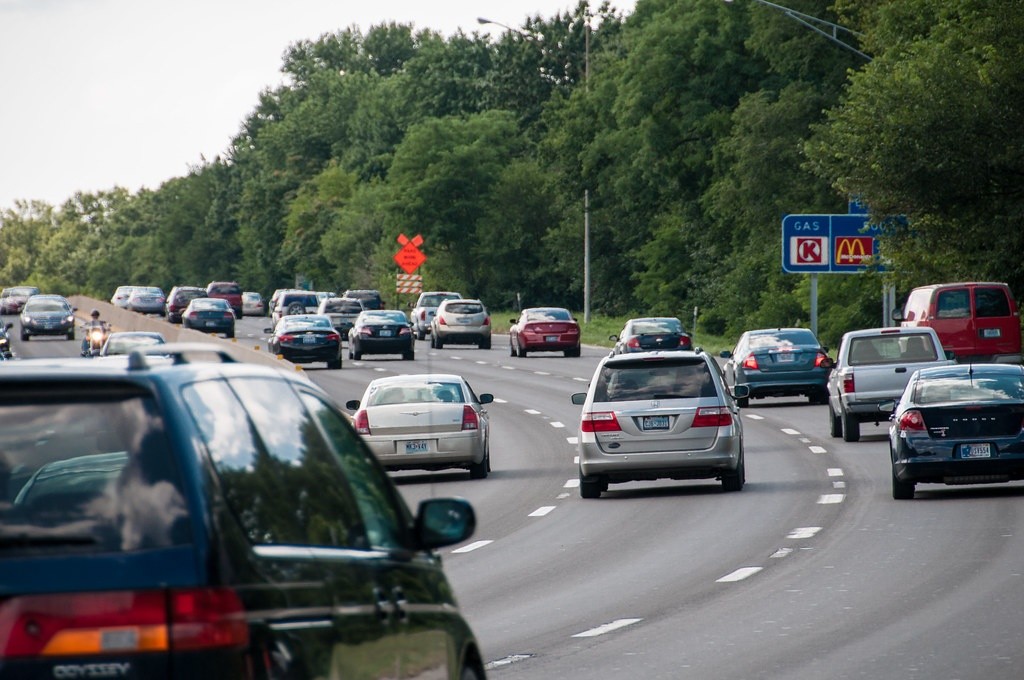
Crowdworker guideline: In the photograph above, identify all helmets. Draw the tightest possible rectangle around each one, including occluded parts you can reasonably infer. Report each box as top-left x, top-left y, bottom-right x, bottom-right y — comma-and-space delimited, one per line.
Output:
91, 310, 100, 316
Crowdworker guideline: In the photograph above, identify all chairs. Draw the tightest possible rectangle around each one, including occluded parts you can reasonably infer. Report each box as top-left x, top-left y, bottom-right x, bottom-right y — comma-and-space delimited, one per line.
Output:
606, 371, 638, 398
900, 337, 931, 359
992, 379, 1018, 400
673, 369, 698, 390
436, 390, 453, 402
852, 342, 882, 360
382, 391, 402, 404
924, 385, 951, 402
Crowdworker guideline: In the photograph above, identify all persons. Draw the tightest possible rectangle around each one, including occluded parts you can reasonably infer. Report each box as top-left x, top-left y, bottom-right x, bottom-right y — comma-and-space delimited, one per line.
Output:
381, 387, 404, 404
433, 387, 453, 402
80, 311, 111, 357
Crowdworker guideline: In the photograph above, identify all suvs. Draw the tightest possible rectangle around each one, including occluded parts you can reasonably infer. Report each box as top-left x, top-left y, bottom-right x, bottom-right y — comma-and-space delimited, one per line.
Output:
570, 347, 750, 498
820, 326, 956, 442
0, 344, 487, 680
891, 280, 1021, 365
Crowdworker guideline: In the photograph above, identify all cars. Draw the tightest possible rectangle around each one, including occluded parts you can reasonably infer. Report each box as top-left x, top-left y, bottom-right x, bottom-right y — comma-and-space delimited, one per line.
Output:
878, 364, 1024, 499
718, 327, 835, 407
509, 307, 582, 358
608, 317, 694, 355
0, 280, 493, 370
345, 373, 495, 480
